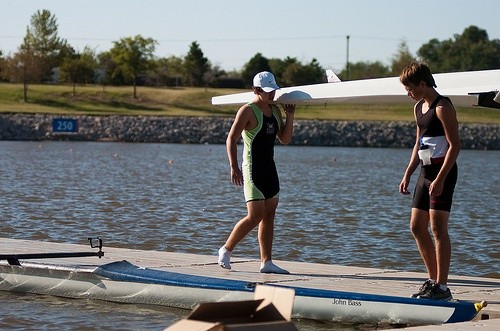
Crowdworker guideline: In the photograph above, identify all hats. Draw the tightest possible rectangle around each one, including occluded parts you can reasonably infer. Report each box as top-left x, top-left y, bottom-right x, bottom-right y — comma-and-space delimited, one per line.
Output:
253, 71, 279, 93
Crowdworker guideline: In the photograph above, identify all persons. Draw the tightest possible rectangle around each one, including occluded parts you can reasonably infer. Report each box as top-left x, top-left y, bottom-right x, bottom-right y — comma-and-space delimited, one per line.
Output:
399, 60, 460, 302
218, 71, 297, 273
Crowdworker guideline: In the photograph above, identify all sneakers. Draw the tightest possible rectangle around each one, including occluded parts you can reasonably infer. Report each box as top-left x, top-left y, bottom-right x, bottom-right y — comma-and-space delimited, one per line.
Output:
417, 284, 453, 301
410, 279, 437, 298
